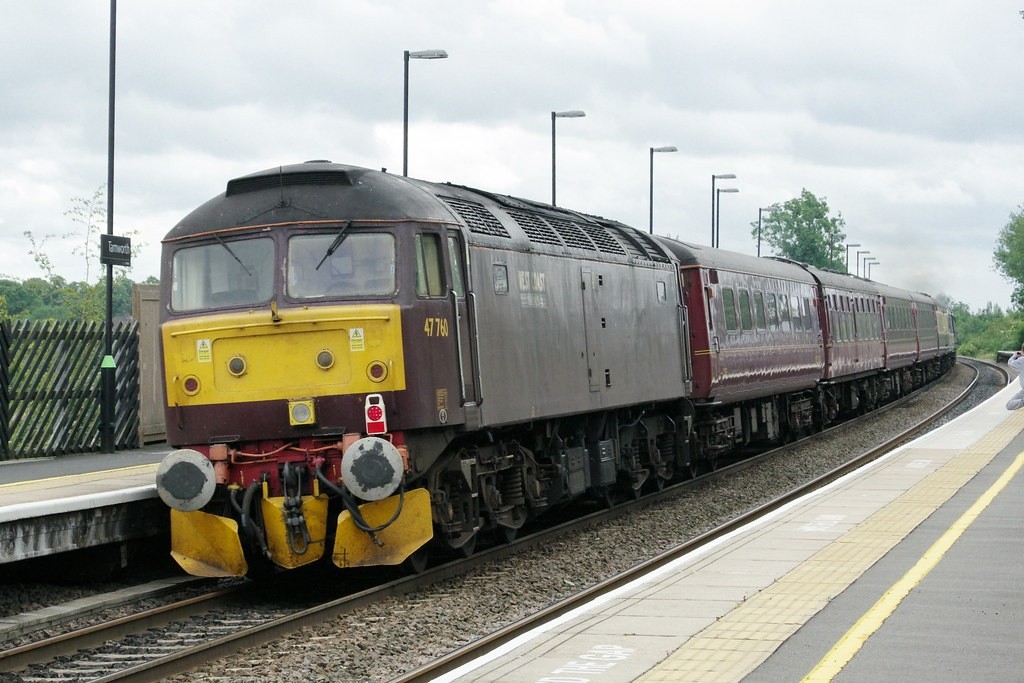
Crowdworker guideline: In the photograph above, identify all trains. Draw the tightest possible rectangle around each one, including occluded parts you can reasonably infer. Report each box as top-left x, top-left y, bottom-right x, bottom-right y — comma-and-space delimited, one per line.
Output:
155, 160, 959, 579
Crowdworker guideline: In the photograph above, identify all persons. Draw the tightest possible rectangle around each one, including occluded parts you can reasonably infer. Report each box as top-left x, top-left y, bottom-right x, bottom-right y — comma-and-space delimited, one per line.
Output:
1006, 343, 1024, 410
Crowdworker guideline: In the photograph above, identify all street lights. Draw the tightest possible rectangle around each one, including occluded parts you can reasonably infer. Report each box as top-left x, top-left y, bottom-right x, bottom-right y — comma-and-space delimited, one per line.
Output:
868, 263, 880, 280
715, 188, 740, 248
403, 48, 449, 179
712, 174, 737, 249
551, 110, 585, 207
649, 146, 680, 233
857, 251, 870, 276
845, 244, 861, 273
758, 208, 781, 257
863, 258, 875, 278
